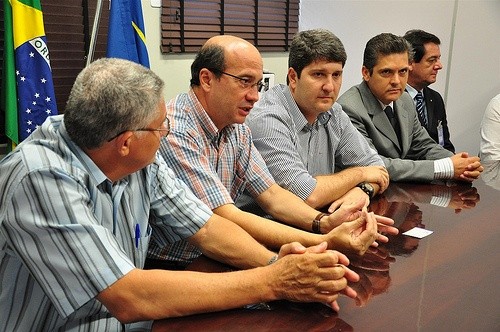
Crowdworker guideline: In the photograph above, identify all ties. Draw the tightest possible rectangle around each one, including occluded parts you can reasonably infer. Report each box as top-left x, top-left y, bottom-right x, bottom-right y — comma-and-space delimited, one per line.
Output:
384, 106, 402, 148
416, 92, 428, 127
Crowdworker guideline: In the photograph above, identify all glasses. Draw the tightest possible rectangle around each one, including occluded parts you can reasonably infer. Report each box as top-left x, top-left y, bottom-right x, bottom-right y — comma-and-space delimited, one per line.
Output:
222, 72, 264, 93
107, 117, 171, 143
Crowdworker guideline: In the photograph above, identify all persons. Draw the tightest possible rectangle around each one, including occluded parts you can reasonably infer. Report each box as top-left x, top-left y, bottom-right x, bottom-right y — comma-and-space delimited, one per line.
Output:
367, 193, 425, 256
332, 240, 391, 306
245, 27, 389, 212
0, 57, 360, 331
336, 32, 484, 182
480, 93, 499, 159
146, 34, 399, 269
394, 180, 480, 215
479, 159, 500, 191
403, 29, 455, 154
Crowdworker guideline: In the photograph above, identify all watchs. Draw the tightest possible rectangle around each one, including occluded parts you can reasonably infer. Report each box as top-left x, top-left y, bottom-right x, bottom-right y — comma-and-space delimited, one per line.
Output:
312, 212, 329, 233
355, 181, 374, 200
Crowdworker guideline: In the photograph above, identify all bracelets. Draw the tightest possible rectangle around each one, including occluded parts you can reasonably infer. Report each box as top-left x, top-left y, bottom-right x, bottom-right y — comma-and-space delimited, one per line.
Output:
269, 254, 277, 265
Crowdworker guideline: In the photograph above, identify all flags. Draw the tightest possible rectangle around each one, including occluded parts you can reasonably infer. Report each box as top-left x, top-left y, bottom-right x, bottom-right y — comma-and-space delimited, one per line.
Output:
0, 0, 58, 154
107, 0, 150, 68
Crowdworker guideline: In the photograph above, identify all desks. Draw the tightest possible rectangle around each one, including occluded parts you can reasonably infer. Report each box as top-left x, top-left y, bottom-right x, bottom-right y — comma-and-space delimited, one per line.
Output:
150, 161, 500, 332
